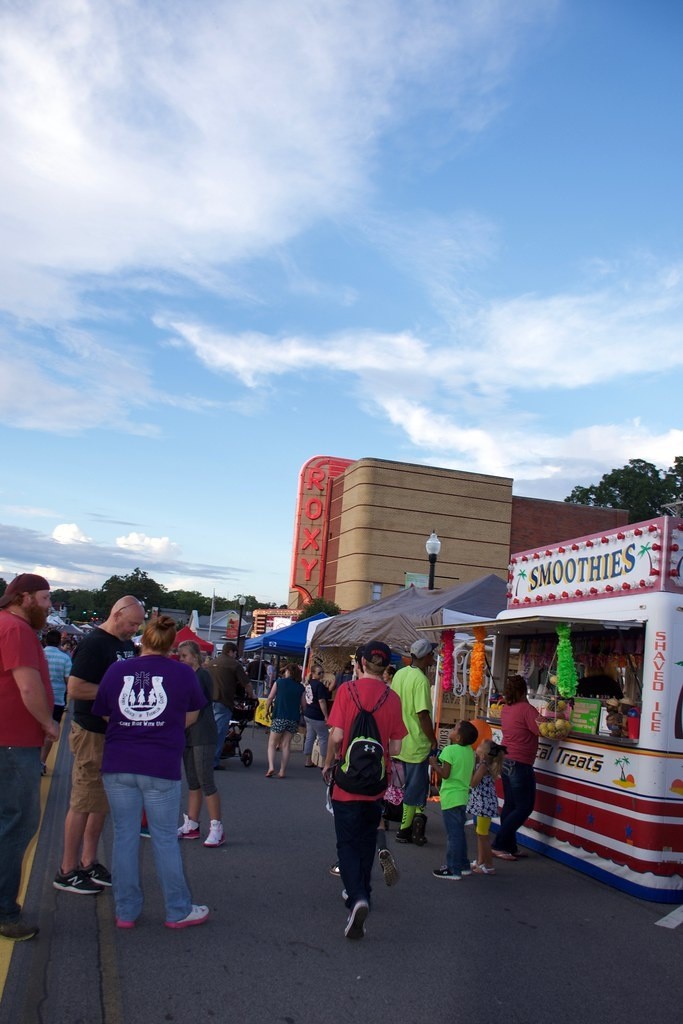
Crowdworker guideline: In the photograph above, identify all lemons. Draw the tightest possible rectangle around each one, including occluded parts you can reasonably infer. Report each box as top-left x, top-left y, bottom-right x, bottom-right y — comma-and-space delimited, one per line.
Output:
491, 704, 504, 710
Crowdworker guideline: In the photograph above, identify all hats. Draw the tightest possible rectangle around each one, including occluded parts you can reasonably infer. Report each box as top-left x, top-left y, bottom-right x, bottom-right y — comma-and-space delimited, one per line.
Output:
0, 572, 50, 608
363, 640, 391, 666
410, 639, 438, 659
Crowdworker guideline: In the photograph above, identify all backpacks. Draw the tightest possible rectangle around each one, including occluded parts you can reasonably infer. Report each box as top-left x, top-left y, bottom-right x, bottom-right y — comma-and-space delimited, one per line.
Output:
335, 681, 390, 796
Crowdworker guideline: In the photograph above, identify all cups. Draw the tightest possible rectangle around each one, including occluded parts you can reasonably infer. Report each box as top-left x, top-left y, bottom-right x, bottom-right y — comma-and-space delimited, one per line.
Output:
627, 716, 641, 739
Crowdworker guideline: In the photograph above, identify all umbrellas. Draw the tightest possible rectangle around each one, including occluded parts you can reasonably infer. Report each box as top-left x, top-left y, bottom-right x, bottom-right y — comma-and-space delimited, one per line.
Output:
45, 615, 70, 627
169, 626, 215, 654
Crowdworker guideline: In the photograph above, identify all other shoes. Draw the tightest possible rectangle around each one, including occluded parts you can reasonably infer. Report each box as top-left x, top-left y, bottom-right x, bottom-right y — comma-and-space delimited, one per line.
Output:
114, 915, 135, 928
329, 860, 341, 877
265, 768, 276, 778
304, 762, 315, 768
214, 765, 226, 771
139, 826, 151, 838
40, 762, 47, 776
277, 772, 286, 778
342, 889, 348, 900
412, 813, 427, 846
378, 847, 400, 887
164, 904, 209, 929
344, 899, 370, 938
377, 828, 391, 854
396, 825, 427, 843
432, 868, 462, 881
439, 864, 472, 876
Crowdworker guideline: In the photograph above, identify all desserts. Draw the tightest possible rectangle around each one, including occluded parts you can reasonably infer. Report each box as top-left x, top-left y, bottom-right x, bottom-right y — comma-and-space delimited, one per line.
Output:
606, 698, 636, 737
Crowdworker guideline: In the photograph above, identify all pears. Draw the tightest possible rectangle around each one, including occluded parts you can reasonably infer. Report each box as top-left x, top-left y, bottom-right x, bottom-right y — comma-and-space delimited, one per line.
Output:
550, 677, 557, 685
539, 719, 570, 739
545, 701, 566, 711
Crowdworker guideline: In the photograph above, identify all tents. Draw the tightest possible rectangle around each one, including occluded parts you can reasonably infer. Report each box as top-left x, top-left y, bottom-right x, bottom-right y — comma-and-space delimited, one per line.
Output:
243, 611, 330, 695
301, 575, 511, 784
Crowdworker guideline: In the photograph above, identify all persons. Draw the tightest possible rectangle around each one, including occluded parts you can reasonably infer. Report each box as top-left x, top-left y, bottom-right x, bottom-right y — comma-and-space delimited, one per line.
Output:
89, 614, 212, 931
38, 629, 80, 776
53, 593, 146, 900
141, 641, 553, 939
0, 573, 60, 941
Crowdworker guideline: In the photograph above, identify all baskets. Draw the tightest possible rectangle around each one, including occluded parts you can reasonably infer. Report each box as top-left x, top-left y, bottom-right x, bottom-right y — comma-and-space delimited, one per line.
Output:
535, 716, 573, 740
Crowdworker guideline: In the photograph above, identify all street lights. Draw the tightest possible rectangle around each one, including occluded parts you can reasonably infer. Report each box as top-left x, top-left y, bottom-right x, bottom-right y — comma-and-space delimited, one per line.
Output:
235, 594, 247, 658
425, 528, 442, 590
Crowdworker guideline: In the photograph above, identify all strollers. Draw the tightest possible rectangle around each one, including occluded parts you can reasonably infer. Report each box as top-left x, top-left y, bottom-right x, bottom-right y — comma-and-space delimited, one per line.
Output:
221, 696, 260, 767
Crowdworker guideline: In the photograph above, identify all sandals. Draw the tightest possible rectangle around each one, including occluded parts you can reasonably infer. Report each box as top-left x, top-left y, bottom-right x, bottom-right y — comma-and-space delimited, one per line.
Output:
470, 860, 497, 874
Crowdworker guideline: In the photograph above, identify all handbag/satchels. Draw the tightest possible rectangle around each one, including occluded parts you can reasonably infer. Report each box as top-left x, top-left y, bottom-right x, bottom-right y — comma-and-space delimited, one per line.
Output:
382, 785, 405, 822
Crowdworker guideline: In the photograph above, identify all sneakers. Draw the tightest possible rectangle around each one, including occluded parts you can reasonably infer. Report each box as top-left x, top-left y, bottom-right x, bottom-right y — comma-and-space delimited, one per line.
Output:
177, 813, 200, 839
0, 914, 41, 942
78, 860, 113, 886
52, 866, 104, 895
203, 819, 226, 847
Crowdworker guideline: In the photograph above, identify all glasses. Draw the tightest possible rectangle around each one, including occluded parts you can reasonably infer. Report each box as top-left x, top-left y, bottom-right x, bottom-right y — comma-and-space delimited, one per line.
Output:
318, 671, 324, 675
119, 600, 145, 611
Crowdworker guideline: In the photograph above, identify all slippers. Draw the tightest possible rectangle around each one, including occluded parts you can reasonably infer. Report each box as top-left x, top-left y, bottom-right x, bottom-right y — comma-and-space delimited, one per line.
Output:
490, 848, 528, 861
